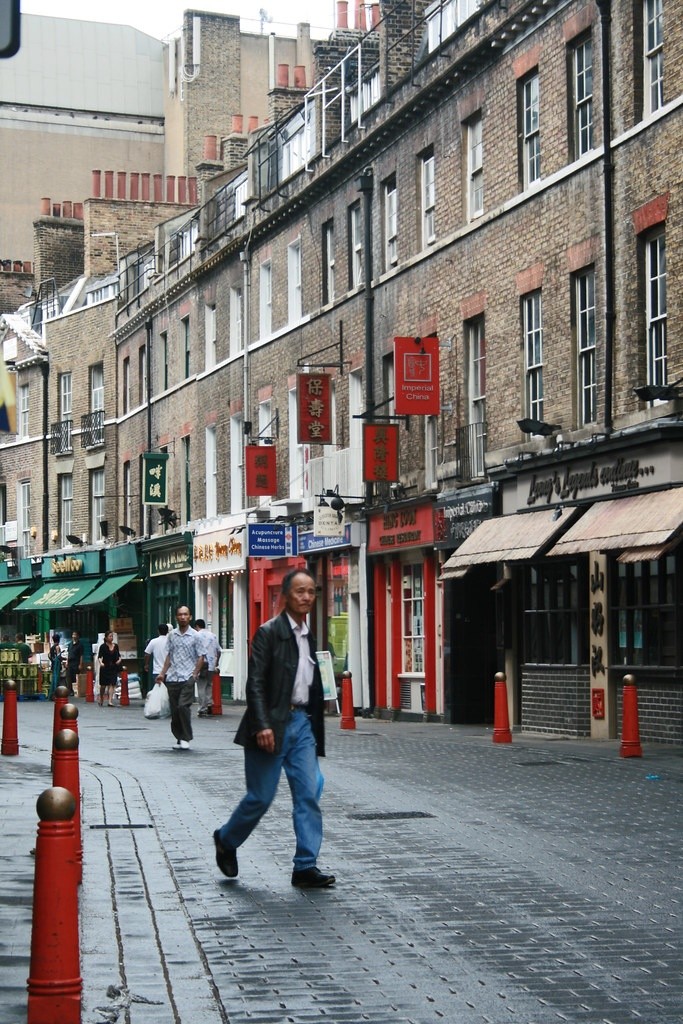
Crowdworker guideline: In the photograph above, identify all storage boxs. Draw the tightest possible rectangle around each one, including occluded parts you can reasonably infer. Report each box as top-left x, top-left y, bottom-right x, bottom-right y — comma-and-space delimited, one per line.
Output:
31, 629, 69, 670
110, 617, 139, 658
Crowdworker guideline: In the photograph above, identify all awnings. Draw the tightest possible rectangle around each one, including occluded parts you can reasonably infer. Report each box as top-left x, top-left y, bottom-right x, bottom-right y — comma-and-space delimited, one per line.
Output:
546, 486, 683, 563
74, 572, 139, 605
436, 507, 580, 579
0, 585, 31, 610
13, 578, 102, 610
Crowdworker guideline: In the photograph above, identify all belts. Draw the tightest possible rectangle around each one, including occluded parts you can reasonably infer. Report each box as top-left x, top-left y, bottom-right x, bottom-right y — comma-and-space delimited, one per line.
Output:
289, 703, 298, 711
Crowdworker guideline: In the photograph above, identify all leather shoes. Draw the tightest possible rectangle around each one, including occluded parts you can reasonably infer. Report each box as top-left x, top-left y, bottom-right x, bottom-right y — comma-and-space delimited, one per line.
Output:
292, 867, 335, 887
108, 704, 115, 707
214, 828, 238, 877
98, 698, 103, 707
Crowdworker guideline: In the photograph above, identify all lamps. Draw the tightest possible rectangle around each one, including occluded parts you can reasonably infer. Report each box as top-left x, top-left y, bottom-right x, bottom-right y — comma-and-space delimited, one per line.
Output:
634, 384, 678, 402
65, 534, 84, 545
157, 507, 181, 526
119, 525, 137, 537
516, 417, 558, 435
0, 545, 13, 554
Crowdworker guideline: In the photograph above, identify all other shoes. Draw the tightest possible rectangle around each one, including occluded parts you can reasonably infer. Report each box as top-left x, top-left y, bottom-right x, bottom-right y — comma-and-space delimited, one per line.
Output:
171, 739, 190, 750
196, 704, 213, 717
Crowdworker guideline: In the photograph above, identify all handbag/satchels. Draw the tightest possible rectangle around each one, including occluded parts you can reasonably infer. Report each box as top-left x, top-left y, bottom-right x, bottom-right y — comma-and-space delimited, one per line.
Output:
144, 680, 172, 719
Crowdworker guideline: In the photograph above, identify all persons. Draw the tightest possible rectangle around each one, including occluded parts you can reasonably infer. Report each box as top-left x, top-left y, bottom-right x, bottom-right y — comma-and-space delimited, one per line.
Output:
156, 605, 209, 749
0, 633, 33, 664
214, 570, 336, 886
48, 634, 65, 687
65, 630, 84, 696
144, 624, 173, 687
98, 630, 122, 707
194, 619, 221, 715
